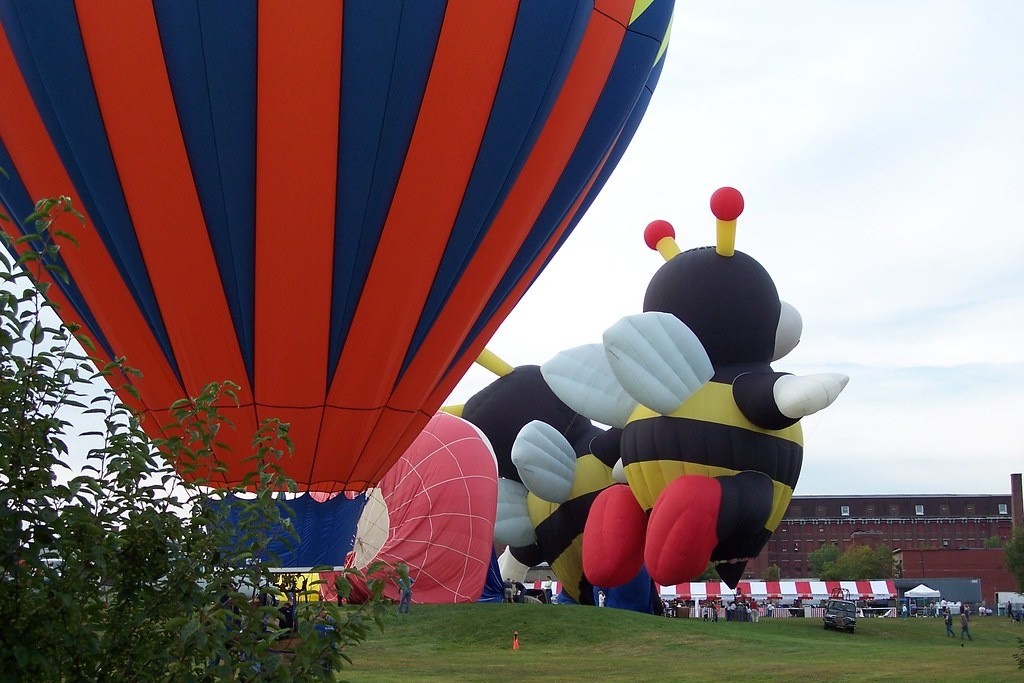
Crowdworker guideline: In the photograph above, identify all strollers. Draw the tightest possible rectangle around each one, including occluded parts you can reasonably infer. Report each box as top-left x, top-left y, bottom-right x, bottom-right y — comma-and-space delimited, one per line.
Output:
1011, 611, 1023, 624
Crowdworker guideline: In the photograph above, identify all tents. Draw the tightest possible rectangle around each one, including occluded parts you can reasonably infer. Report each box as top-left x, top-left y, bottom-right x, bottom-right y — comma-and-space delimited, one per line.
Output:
905, 584, 940, 615
523, 581, 898, 600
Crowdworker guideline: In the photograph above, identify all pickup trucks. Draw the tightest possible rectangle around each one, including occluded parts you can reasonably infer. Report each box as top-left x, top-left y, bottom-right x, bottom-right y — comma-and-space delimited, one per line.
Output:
823, 599, 861, 633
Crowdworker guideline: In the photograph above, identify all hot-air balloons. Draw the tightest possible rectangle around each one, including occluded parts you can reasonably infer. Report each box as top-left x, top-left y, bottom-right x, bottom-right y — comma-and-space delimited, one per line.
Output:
1, 0, 676, 671
538, 186, 851, 589
439, 347, 668, 616
274, 410, 552, 604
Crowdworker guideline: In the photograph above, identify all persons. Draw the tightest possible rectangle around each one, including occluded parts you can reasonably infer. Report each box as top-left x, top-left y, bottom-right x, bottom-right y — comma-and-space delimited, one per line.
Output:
903, 604, 907, 619
335, 575, 345, 607
1019, 604, 1024, 624
397, 573, 415, 614
852, 598, 868, 608
504, 578, 527, 603
661, 598, 720, 622
979, 605, 992, 617
209, 572, 339, 680
545, 576, 552, 603
911, 600, 937, 617
1008, 600, 1015, 617
794, 598, 803, 617
941, 598, 955, 636
960, 602, 972, 640
725, 598, 772, 622
598, 591, 605, 607
